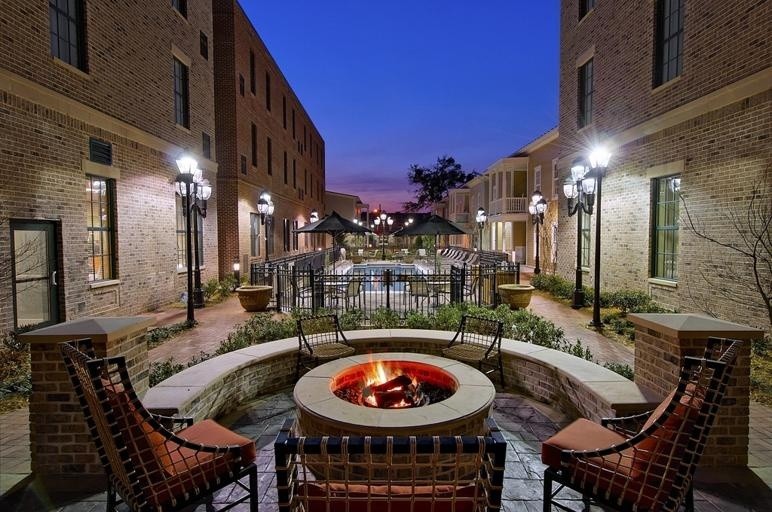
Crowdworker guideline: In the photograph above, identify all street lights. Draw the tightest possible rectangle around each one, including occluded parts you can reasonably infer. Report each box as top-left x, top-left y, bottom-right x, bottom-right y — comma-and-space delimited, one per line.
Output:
589, 145, 612, 327
256, 190, 275, 261
174, 147, 213, 324
529, 190, 548, 274
563, 156, 596, 309
476, 208, 487, 250
374, 210, 394, 260
310, 209, 319, 251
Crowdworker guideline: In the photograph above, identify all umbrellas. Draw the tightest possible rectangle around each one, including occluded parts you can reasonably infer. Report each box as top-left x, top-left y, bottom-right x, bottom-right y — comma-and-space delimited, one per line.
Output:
391, 213, 467, 282
290, 210, 372, 294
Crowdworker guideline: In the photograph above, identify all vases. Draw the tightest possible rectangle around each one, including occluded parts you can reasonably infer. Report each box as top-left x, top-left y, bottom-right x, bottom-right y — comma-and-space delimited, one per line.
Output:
497, 283, 535, 310
235, 286, 273, 310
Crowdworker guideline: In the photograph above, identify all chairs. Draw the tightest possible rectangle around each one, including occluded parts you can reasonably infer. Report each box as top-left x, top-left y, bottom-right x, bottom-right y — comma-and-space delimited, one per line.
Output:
290, 276, 363, 310
408, 265, 479, 311
